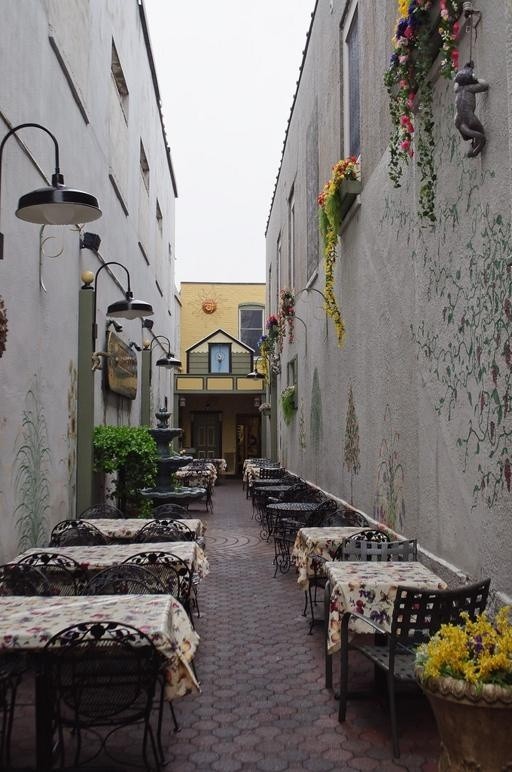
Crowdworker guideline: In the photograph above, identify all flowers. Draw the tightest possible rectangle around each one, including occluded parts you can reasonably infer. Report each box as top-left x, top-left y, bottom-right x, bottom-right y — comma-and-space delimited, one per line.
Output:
418, 593, 512, 694
377, 0, 468, 227
316, 158, 359, 346
258, 288, 296, 357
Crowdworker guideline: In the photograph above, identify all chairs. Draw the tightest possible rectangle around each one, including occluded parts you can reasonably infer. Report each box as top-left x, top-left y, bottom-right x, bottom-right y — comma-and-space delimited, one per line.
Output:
242, 457, 369, 579
324, 537, 418, 689
174, 458, 227, 514
0, 504, 211, 630
35, 621, 181, 771
0, 648, 31, 771
302, 530, 392, 634
338, 576, 492, 760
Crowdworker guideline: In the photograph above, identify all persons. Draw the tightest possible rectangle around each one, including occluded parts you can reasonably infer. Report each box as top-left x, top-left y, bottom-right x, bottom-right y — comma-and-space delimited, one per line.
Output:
247, 434, 259, 459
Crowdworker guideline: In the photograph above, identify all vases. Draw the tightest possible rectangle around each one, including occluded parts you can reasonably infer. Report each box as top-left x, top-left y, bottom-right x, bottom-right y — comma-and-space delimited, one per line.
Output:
334, 178, 361, 224
405, 0, 452, 84
422, 662, 512, 772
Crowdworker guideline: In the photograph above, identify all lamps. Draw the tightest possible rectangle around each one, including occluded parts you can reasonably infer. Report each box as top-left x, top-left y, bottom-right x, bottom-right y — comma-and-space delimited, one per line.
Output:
1, 119, 105, 228
180, 395, 186, 407
92, 259, 155, 351
141, 318, 154, 330
150, 335, 182, 385
80, 231, 103, 251
246, 355, 272, 395
253, 395, 261, 408
